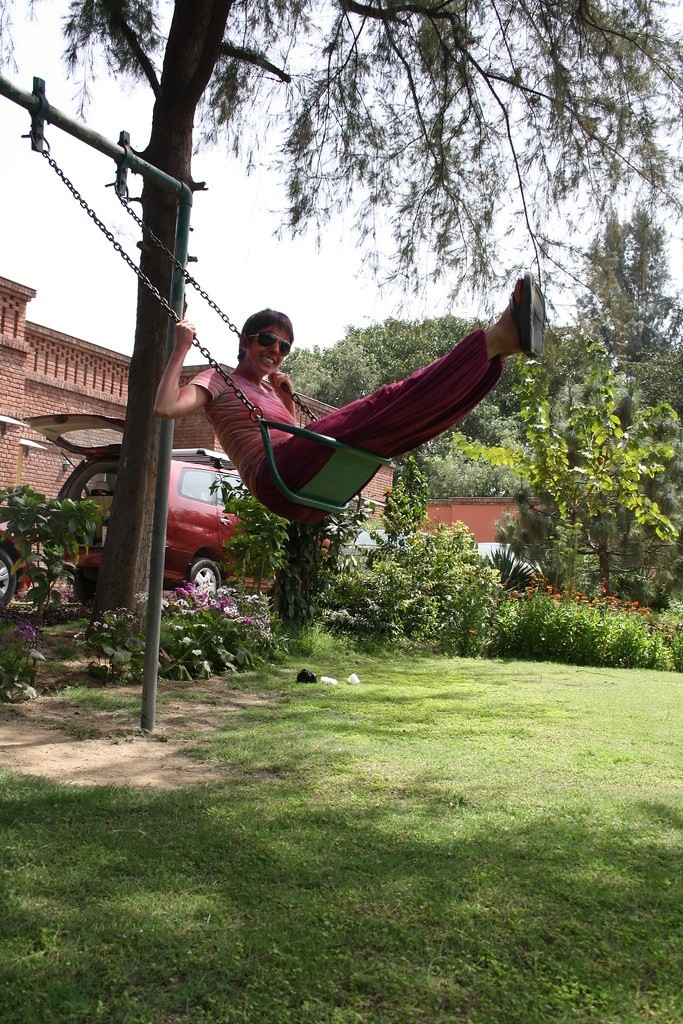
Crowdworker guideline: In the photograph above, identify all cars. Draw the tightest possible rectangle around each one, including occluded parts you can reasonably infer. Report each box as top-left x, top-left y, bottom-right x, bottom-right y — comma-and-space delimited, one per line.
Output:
337, 530, 437, 599
0, 504, 34, 612
22, 414, 333, 604
443, 543, 546, 601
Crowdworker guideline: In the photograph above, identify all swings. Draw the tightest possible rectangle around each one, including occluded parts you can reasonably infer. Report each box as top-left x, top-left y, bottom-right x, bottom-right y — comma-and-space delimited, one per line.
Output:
31, 141, 389, 515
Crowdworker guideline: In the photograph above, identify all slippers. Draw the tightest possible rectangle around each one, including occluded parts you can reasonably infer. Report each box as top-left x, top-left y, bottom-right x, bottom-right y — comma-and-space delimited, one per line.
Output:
509, 273, 546, 358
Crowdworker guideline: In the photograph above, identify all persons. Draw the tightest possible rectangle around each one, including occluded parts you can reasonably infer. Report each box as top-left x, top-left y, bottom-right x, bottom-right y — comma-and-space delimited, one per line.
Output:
154, 272, 546, 522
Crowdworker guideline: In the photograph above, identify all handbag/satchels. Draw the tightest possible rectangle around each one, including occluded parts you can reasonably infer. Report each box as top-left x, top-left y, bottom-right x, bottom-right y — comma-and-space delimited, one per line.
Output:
297, 668, 317, 685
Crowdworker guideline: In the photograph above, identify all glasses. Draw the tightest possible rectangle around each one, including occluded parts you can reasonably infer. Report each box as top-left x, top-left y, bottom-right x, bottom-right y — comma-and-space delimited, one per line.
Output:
249, 331, 292, 357
